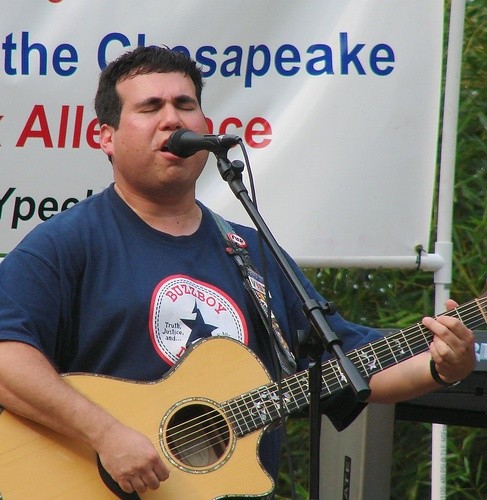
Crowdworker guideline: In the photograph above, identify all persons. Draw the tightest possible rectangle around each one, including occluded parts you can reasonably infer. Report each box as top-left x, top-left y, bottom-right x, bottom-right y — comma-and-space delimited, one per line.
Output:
0, 44, 476, 500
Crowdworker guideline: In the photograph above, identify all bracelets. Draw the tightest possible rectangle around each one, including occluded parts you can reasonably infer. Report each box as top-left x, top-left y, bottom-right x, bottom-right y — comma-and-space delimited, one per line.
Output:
430, 356, 463, 389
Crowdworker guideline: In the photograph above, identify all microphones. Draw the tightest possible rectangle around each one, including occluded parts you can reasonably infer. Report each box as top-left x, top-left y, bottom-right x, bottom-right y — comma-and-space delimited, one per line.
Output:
167, 129, 242, 159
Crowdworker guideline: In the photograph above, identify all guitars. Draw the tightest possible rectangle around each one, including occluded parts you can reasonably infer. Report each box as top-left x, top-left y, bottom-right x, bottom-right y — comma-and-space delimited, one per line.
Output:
0, 290, 487, 500
